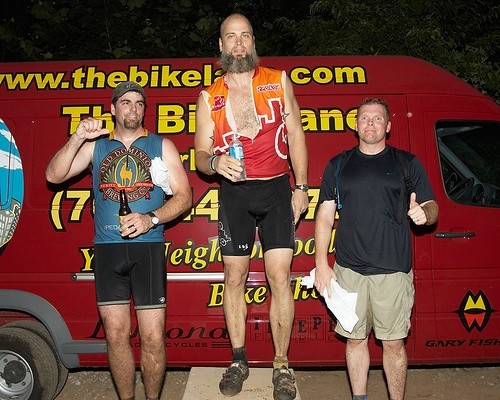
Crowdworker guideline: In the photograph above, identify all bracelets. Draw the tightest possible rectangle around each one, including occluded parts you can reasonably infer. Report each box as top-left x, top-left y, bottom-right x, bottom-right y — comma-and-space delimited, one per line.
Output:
210, 155, 219, 173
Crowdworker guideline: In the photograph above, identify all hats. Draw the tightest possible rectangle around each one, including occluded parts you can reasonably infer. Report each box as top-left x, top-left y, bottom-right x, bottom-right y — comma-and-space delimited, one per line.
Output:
112, 82, 146, 102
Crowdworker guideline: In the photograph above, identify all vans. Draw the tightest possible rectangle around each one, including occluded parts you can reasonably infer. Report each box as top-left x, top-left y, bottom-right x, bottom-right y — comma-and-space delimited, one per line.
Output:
1, 56, 500, 400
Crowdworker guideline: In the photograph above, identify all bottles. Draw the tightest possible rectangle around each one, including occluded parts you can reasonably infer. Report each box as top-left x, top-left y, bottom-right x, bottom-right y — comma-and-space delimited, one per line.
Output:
119, 189, 134, 239
230, 133, 245, 182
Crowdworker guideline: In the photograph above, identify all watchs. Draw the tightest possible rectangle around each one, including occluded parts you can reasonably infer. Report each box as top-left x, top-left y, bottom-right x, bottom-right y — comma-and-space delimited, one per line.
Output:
295, 184, 309, 192
146, 212, 159, 228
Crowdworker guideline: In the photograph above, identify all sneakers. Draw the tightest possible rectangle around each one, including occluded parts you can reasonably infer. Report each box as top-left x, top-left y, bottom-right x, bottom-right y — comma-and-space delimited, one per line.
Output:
219, 360, 249, 397
272, 365, 296, 400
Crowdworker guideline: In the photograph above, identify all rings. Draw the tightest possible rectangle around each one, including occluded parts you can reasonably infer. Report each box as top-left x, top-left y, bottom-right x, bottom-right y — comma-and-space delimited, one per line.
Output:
416, 217, 419, 221
133, 226, 137, 230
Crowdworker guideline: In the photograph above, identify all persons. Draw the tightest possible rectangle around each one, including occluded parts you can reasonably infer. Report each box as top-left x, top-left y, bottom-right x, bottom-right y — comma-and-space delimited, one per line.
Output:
45, 81, 193, 400
195, 14, 309, 400
315, 98, 438, 400
